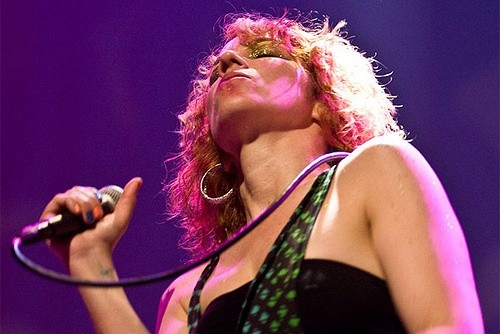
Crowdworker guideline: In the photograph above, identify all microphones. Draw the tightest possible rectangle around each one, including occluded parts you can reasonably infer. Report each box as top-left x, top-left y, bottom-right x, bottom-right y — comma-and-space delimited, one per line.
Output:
17, 185, 125, 242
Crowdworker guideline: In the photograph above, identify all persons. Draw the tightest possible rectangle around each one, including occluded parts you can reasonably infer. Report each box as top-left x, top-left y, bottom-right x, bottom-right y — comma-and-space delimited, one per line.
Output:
40, 6, 488, 334
